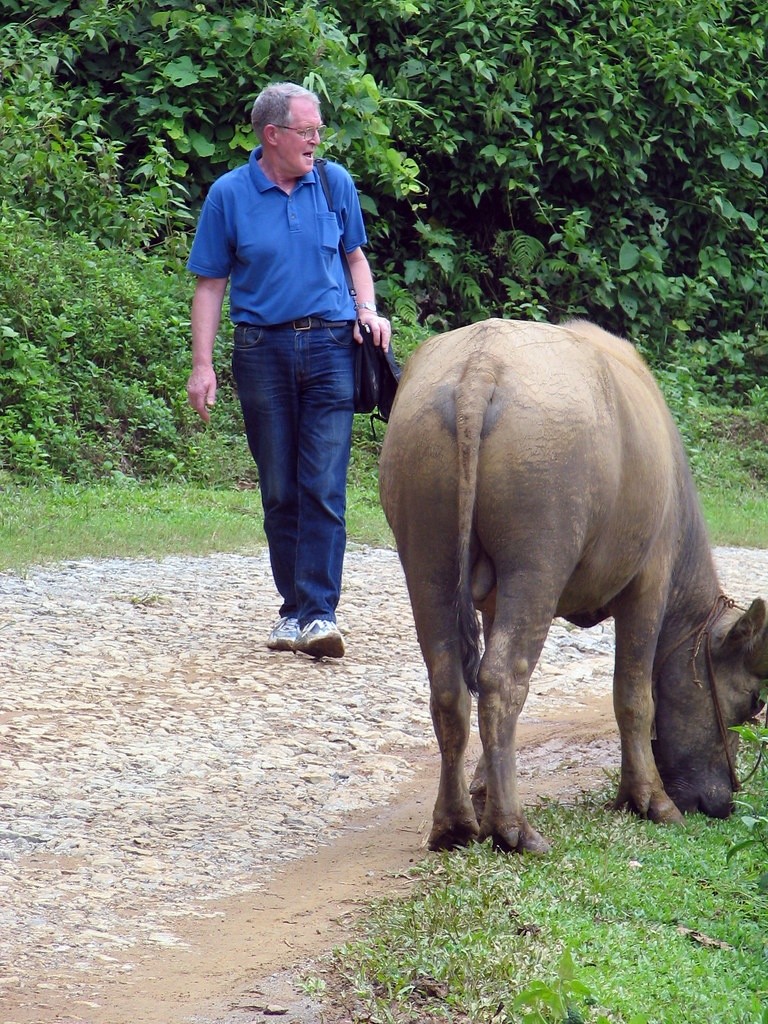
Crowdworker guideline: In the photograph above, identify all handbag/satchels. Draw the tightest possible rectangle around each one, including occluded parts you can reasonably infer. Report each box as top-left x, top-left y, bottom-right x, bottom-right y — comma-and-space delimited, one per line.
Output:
354, 319, 404, 441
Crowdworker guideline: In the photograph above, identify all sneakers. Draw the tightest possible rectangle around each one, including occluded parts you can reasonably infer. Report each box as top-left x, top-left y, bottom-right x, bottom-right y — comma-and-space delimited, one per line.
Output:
267, 616, 302, 651
294, 618, 346, 658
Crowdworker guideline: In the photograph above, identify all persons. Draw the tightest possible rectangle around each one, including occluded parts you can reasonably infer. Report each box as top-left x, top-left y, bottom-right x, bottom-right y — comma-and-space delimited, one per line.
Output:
186, 83, 392, 659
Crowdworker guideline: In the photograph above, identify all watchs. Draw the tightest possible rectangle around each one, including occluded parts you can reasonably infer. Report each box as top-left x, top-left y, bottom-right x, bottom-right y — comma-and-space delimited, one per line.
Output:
358, 302, 377, 312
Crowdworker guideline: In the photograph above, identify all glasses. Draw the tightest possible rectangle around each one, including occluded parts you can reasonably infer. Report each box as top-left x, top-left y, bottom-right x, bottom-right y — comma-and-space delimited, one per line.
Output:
268, 122, 328, 142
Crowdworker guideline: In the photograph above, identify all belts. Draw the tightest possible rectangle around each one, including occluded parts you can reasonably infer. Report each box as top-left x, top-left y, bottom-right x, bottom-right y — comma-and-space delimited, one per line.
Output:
260, 316, 348, 332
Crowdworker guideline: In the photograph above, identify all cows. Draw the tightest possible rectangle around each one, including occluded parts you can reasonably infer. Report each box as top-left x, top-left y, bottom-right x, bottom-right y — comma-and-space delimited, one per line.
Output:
375, 318, 767, 852
